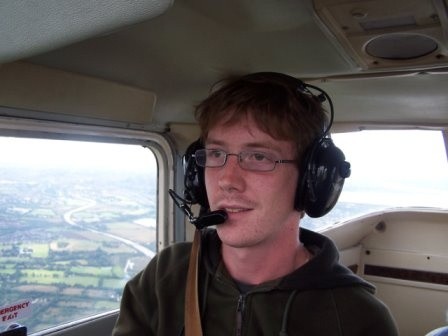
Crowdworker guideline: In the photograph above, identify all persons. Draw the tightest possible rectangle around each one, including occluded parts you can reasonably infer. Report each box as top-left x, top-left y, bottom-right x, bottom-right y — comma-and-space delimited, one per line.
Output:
113, 72, 400, 336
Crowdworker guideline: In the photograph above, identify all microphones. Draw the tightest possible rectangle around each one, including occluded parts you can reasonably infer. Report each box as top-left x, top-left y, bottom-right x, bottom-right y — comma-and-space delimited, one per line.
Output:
168, 189, 228, 231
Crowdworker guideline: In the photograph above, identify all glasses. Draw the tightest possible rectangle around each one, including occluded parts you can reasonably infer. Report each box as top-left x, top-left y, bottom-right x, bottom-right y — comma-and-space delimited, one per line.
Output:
192, 148, 302, 172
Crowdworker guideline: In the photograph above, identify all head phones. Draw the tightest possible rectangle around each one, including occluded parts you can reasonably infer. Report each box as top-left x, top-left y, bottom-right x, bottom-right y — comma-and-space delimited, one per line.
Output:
182, 71, 351, 219
